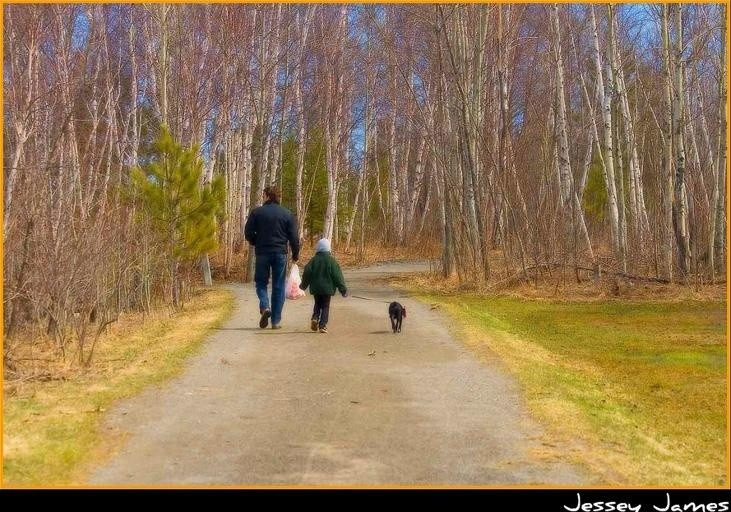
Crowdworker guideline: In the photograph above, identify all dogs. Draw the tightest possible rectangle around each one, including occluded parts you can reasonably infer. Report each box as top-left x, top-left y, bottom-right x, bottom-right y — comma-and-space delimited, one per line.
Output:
388, 301, 406, 333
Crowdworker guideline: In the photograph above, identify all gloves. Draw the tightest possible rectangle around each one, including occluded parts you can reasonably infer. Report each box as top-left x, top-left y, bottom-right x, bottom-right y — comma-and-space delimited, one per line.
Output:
342, 290, 348, 297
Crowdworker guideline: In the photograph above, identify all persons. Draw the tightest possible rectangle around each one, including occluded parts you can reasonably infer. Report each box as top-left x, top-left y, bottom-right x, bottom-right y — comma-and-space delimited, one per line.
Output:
244, 185, 299, 330
299, 238, 348, 333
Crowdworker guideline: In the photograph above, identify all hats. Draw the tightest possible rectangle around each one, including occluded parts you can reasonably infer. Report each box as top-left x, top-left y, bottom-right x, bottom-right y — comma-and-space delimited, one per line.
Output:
317, 238, 331, 252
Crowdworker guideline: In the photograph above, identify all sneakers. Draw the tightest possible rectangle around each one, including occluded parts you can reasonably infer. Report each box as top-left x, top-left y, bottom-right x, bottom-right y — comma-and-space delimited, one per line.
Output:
260, 308, 273, 328
320, 325, 328, 332
312, 319, 319, 330
273, 320, 282, 328
